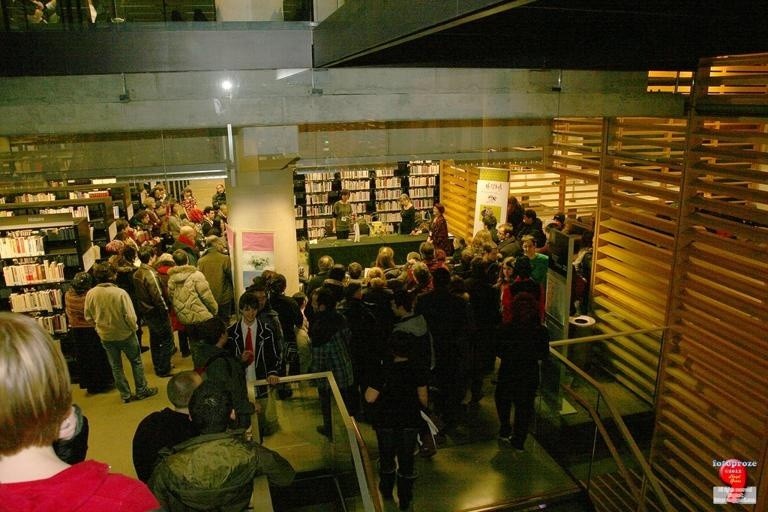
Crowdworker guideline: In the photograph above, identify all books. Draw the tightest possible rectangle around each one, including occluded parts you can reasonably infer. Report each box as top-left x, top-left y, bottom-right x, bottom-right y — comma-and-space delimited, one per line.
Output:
304, 160, 440, 237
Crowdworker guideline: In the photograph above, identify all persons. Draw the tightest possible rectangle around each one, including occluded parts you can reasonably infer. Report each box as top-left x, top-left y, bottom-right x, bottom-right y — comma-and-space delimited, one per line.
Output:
25, 0, 99, 24
1, 184, 595, 511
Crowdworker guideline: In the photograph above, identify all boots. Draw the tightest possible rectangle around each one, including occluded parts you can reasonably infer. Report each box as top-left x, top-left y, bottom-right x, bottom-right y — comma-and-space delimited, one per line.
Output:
376, 459, 418, 509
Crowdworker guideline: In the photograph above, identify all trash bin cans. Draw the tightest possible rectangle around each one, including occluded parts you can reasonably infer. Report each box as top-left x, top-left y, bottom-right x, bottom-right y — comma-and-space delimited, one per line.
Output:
569, 315, 596, 367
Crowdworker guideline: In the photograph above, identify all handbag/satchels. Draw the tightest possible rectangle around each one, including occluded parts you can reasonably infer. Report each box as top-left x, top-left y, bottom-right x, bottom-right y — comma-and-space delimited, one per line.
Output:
284, 342, 300, 364
418, 413, 441, 457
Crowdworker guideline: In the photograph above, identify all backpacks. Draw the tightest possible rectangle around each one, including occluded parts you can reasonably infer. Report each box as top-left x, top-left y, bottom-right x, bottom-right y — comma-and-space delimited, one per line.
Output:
129, 408, 189, 481
193, 355, 232, 381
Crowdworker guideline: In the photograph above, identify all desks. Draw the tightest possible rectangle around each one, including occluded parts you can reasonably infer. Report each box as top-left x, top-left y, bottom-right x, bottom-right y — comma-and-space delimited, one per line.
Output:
304, 232, 453, 274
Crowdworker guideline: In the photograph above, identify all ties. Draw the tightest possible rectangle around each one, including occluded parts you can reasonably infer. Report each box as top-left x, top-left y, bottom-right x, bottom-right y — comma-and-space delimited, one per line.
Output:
244, 328, 255, 365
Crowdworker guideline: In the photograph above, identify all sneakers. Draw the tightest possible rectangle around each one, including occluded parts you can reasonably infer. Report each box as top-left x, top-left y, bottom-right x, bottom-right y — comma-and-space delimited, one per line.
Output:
122, 388, 158, 402
160, 367, 181, 377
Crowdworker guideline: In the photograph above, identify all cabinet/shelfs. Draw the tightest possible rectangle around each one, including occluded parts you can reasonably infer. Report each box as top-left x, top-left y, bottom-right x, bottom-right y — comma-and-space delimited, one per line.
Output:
292, 159, 440, 239
1, 179, 157, 338
